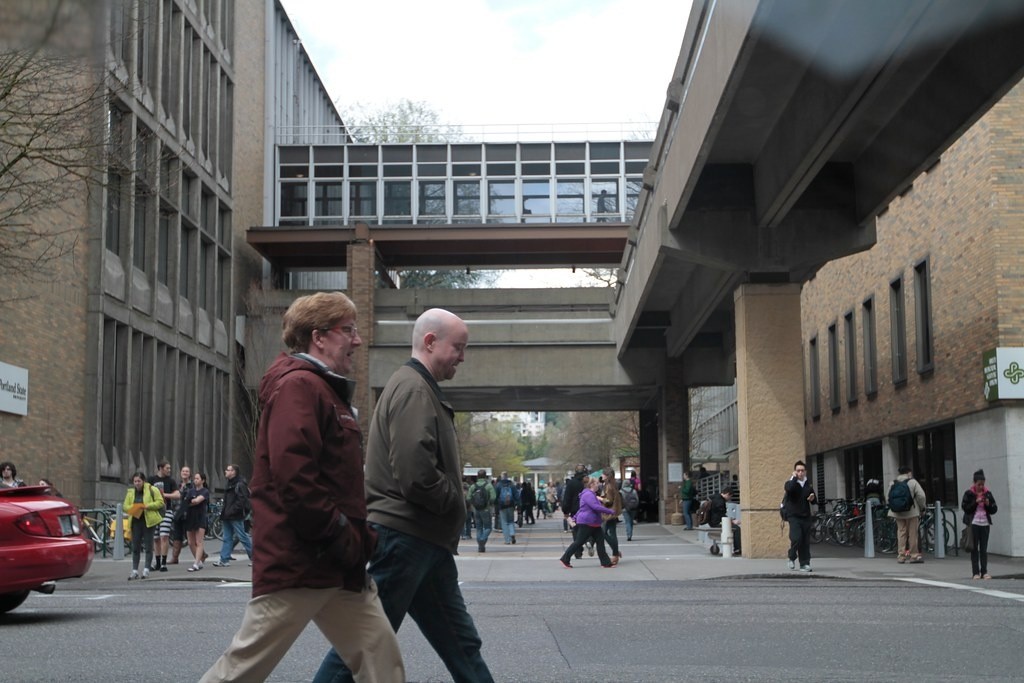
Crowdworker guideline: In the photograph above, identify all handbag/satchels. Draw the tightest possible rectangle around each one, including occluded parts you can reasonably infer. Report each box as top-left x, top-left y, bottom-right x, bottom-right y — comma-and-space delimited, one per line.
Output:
149, 484, 167, 517
960, 525, 974, 553
684, 482, 699, 498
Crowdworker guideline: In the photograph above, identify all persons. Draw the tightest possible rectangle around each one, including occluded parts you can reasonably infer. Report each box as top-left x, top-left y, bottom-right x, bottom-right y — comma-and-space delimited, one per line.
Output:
886, 465, 927, 563
39, 479, 62, 498
198, 291, 406, 683
122, 471, 164, 580
677, 466, 741, 554
461, 463, 659, 569
961, 469, 997, 578
784, 461, 818, 572
0, 462, 28, 490
148, 461, 180, 571
166, 461, 255, 572
312, 307, 495, 683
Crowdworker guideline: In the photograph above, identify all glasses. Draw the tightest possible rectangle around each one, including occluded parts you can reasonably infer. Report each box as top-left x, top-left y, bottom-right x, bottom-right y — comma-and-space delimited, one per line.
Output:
322, 323, 358, 337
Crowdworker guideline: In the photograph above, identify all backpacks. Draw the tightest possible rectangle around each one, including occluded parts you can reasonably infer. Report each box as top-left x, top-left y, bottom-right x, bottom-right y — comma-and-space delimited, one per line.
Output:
471, 482, 489, 510
498, 480, 513, 506
779, 493, 789, 529
621, 488, 638, 514
887, 477, 917, 513
234, 481, 252, 518
695, 497, 711, 525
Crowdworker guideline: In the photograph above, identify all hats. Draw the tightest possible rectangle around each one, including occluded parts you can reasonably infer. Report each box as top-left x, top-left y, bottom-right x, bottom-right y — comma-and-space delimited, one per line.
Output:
898, 464, 912, 474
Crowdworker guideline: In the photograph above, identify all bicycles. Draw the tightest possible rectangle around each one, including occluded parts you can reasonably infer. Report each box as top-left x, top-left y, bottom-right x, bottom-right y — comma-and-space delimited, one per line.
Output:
77, 497, 254, 556
810, 497, 950, 553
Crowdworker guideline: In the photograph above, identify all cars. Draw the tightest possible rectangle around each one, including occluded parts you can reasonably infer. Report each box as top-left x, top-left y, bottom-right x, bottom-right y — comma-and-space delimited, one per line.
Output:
0, 484, 96, 615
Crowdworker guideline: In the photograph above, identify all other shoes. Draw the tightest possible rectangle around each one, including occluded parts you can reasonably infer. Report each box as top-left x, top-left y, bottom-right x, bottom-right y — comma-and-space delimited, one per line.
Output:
492, 527, 502, 533
587, 543, 594, 557
612, 551, 622, 565
789, 559, 796, 570
982, 574, 992, 579
601, 563, 616, 568
477, 538, 485, 552
127, 553, 252, 580
511, 535, 516, 544
972, 574, 981, 579
799, 564, 812, 572
897, 558, 905, 563
559, 558, 574, 568
683, 527, 693, 530
627, 536, 632, 541
910, 555, 924, 563
